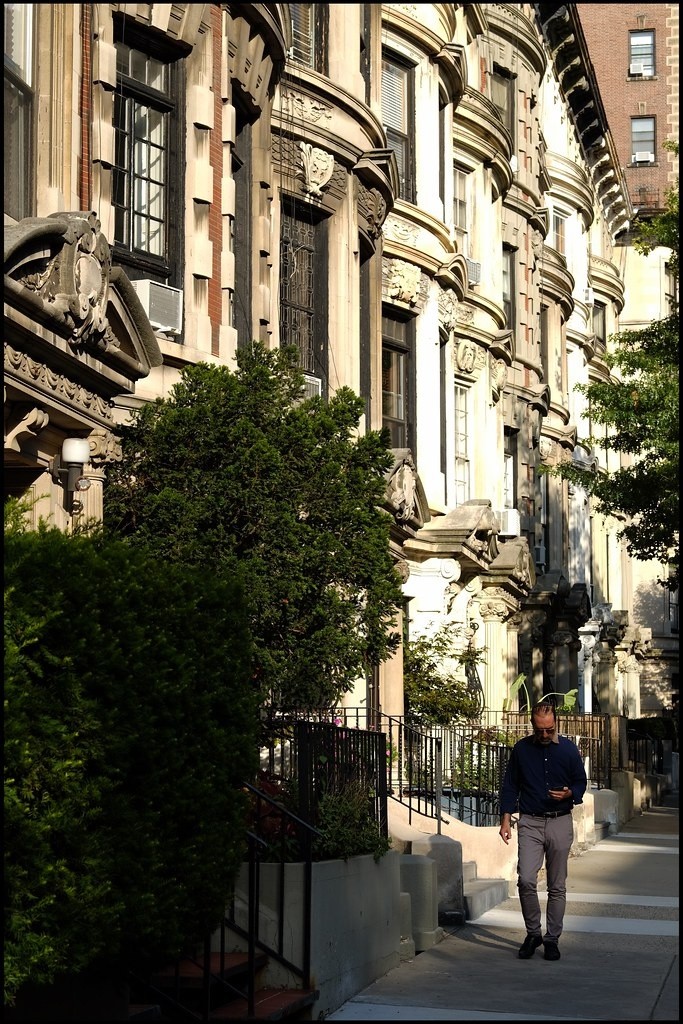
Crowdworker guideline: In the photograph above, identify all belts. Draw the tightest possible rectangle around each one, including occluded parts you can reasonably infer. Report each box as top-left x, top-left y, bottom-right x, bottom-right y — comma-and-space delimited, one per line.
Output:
523, 810, 571, 819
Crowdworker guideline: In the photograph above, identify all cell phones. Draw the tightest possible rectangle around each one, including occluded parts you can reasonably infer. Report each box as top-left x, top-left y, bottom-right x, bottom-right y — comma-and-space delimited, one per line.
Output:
552, 786, 564, 792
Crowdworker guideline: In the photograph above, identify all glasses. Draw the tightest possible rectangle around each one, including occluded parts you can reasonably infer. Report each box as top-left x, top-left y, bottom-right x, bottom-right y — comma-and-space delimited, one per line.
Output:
532, 723, 555, 735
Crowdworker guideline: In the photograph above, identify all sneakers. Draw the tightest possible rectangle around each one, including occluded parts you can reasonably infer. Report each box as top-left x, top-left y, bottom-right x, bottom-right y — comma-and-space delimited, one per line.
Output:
518, 934, 543, 959
543, 940, 560, 960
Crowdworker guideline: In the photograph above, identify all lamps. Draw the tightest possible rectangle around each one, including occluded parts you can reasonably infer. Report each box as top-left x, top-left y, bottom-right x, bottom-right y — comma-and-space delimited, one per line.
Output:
49, 430, 90, 491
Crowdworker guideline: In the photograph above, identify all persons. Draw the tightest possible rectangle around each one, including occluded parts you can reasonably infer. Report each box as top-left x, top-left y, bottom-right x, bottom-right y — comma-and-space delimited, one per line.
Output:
500, 703, 586, 962
